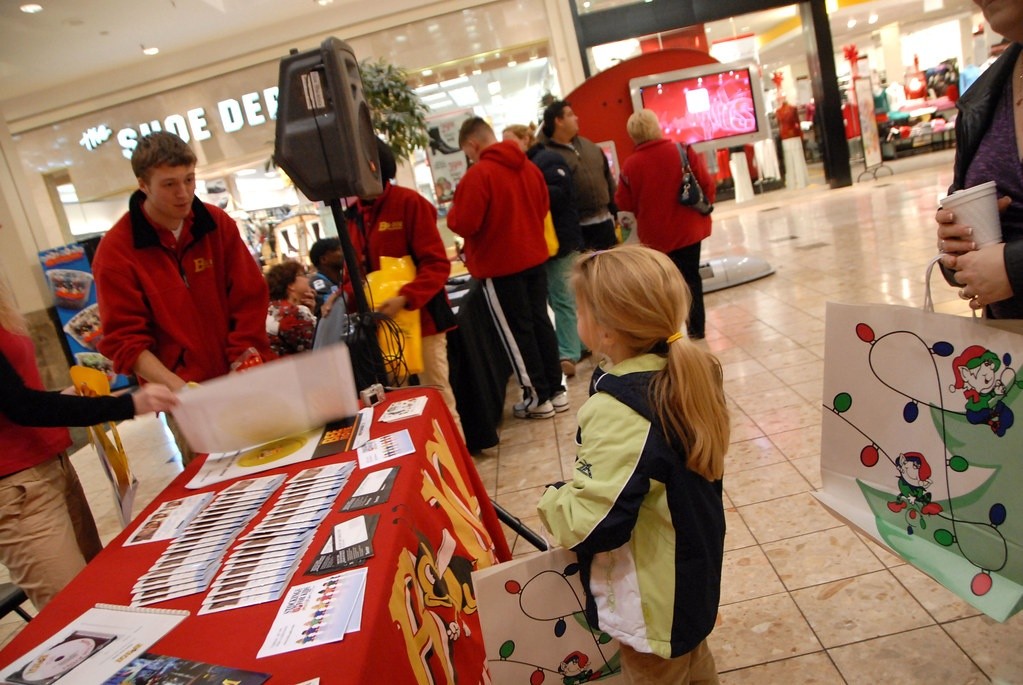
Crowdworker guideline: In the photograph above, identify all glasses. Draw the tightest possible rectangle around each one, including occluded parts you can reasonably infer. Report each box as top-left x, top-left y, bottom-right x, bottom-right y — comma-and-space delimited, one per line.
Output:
295, 270, 307, 279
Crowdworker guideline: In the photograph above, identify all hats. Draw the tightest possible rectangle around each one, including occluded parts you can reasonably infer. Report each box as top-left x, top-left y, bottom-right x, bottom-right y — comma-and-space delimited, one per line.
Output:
307, 236, 342, 263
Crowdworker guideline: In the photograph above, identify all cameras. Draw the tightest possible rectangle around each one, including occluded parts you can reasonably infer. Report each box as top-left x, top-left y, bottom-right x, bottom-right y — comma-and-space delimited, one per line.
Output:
360, 384, 386, 408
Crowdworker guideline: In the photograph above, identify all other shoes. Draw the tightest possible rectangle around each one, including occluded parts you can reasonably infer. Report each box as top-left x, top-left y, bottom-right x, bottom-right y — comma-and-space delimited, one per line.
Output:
560, 360, 575, 376
513, 400, 556, 419
578, 350, 592, 364
551, 392, 570, 413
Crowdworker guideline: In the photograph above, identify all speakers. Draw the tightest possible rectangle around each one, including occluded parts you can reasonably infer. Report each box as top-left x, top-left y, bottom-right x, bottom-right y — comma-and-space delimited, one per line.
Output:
274, 36, 384, 206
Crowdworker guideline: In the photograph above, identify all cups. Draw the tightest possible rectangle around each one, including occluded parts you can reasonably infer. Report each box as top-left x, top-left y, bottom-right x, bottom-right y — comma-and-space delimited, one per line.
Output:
941, 180, 1000, 252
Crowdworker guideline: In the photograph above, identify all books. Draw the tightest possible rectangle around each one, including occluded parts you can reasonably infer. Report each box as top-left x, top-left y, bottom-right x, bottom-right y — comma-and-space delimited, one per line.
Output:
130, 427, 416, 661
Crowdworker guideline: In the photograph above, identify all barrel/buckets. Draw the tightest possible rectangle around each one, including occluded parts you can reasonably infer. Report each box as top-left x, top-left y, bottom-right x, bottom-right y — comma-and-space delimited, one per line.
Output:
45, 269, 94, 308
76, 352, 117, 385
63, 303, 100, 348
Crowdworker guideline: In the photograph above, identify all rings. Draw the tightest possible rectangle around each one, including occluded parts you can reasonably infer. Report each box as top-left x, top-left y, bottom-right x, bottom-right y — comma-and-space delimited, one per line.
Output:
941, 240, 944, 251
975, 299, 984, 310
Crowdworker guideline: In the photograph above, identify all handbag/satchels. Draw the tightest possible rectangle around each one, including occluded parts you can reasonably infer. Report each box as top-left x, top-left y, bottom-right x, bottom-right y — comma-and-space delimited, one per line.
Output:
677, 142, 714, 216
470, 517, 623, 685
809, 256, 1023, 622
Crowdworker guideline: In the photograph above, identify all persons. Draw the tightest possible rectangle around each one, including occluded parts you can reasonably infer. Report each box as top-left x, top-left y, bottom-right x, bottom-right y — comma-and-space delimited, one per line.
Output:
502, 126, 591, 376
872, 77, 928, 115
0, 304, 178, 616
320, 136, 465, 454
96, 131, 270, 396
776, 101, 801, 139
263, 261, 317, 358
306, 238, 345, 305
535, 246, 732, 684
447, 118, 571, 422
615, 110, 715, 341
937, 0, 1023, 320
533, 102, 618, 255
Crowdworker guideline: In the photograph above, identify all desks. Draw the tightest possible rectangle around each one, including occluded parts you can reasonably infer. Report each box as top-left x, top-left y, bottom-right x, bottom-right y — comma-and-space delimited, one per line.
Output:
0, 389, 548, 685
447, 273, 515, 454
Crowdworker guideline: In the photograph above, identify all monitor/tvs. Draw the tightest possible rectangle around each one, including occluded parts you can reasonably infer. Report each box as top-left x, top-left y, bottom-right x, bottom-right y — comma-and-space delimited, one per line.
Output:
628, 58, 771, 153
